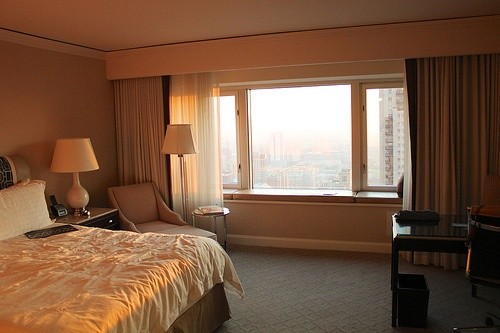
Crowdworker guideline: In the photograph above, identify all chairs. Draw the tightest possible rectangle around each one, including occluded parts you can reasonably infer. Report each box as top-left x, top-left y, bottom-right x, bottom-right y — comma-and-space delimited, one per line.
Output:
471, 175, 500, 218
106, 182, 217, 242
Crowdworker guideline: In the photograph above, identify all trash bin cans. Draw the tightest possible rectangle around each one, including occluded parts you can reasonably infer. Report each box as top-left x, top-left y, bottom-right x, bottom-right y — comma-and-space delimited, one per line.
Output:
396, 272, 430, 330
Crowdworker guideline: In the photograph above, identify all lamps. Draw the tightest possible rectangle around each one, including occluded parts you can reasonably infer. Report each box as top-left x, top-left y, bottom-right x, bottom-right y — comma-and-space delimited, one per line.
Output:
50, 138, 100, 217
160, 124, 197, 222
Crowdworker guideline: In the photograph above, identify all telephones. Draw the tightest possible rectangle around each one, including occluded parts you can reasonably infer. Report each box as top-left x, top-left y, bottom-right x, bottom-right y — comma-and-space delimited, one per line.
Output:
49, 194, 69, 218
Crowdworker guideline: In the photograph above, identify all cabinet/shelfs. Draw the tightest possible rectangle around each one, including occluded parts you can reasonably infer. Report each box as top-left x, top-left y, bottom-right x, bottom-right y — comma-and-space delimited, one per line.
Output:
465, 213, 500, 298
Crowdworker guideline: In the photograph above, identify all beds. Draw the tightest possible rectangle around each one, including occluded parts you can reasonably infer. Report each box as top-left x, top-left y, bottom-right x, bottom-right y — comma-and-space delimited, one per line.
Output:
0, 155, 245, 333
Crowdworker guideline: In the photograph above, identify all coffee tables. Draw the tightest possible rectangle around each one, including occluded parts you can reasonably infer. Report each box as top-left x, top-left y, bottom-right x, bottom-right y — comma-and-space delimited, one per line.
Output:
191, 207, 230, 254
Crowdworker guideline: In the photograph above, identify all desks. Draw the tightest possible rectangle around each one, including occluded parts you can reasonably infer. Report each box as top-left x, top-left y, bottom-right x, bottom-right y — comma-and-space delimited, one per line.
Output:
391, 213, 468, 329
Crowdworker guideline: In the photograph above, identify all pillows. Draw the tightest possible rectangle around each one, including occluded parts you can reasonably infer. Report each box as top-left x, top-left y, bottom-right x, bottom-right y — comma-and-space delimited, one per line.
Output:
0, 178, 56, 242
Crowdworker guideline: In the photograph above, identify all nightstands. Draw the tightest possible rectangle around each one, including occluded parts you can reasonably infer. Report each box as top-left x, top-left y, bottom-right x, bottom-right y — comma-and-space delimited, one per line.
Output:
50, 207, 121, 230
